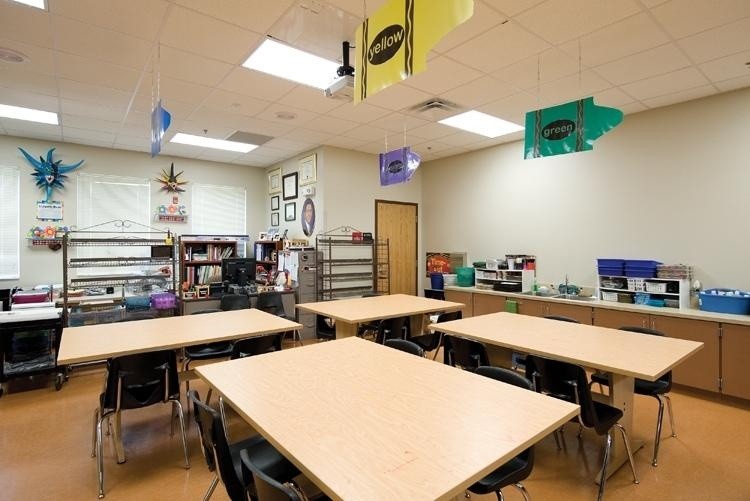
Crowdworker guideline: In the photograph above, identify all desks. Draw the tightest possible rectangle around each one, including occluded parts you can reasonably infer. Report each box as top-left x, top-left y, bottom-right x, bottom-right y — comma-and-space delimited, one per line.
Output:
297, 292, 467, 338
56, 307, 304, 464
428, 309, 706, 485
193, 334, 583, 500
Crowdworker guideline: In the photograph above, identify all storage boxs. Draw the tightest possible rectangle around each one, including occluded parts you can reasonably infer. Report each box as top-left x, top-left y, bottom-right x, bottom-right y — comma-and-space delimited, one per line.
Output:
151, 292, 176, 309
125, 295, 151, 308
697, 287, 750, 314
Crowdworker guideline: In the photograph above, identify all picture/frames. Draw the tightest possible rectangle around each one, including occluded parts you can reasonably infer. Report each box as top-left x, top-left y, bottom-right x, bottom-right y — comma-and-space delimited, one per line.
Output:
282, 171, 298, 199
285, 202, 296, 220
298, 152, 316, 187
267, 167, 283, 195
271, 212, 279, 226
271, 195, 279, 210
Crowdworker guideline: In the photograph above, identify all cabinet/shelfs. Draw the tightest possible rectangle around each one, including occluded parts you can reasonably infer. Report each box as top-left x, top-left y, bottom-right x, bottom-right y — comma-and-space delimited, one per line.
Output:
720, 319, 750, 400
508, 294, 593, 325
597, 274, 691, 310
473, 268, 535, 294
316, 225, 391, 302
253, 240, 284, 288
178, 239, 238, 301
64, 230, 184, 324
277, 248, 323, 338
593, 303, 722, 395
447, 285, 507, 318
9, 285, 54, 308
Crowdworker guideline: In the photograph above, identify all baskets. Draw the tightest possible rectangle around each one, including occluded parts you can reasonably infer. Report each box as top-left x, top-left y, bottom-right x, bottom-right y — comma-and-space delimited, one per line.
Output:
634, 293, 650, 305
602, 292, 618, 301
627, 279, 646, 292
646, 282, 667, 293
618, 294, 632, 303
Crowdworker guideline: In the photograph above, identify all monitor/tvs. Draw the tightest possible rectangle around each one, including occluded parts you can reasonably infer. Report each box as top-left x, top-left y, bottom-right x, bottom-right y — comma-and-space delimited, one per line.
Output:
222, 258, 256, 286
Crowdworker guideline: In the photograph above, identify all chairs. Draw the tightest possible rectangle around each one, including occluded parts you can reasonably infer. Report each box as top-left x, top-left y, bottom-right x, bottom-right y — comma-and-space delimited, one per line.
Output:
230, 333, 282, 360
587, 324, 678, 467
384, 337, 425, 357
191, 389, 303, 500
524, 353, 640, 501
180, 308, 235, 417
442, 332, 491, 371
220, 294, 252, 310
467, 363, 537, 501
361, 293, 385, 336
313, 297, 366, 339
516, 313, 582, 375
406, 310, 463, 360
374, 316, 414, 345
255, 291, 303, 347
89, 348, 192, 500
237, 448, 330, 500
424, 288, 446, 317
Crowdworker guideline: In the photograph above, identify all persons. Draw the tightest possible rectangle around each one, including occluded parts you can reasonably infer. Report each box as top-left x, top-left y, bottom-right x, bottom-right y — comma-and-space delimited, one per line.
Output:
302, 201, 314, 237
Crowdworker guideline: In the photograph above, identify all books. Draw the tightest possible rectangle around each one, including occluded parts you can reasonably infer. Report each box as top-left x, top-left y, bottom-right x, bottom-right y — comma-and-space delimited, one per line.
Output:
208, 244, 235, 262
256, 244, 271, 262
185, 265, 223, 285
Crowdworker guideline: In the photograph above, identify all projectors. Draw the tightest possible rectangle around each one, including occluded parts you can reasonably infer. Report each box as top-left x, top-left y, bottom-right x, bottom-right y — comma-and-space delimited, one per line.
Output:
326, 74, 354, 103
